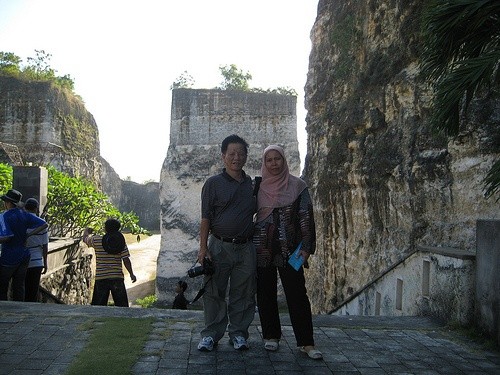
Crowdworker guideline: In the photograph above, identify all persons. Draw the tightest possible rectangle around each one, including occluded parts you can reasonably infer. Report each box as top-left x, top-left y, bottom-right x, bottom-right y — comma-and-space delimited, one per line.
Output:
172, 280, 188, 309
137, 235, 140, 243
253, 145, 324, 359
197, 135, 256, 350
83, 219, 137, 307
0, 190, 49, 302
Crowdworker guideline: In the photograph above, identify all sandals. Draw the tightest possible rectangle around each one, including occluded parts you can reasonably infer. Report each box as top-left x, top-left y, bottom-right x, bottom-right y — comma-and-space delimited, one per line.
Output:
265, 339, 279, 350
300, 346, 322, 359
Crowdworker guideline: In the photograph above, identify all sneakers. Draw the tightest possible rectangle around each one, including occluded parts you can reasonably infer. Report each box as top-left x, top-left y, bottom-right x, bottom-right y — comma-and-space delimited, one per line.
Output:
197, 337, 214, 352
229, 336, 249, 349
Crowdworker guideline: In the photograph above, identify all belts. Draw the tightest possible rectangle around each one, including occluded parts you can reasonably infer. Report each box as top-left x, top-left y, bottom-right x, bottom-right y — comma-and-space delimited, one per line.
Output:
213, 233, 248, 244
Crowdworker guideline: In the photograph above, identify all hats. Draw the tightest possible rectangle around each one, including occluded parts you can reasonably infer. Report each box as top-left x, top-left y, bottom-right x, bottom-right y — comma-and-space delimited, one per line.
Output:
0, 190, 22, 204
102, 232, 126, 254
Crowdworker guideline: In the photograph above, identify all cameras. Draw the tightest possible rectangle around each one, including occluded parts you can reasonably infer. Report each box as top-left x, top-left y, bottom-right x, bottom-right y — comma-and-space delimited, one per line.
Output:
88, 228, 93, 234
188, 258, 215, 278
